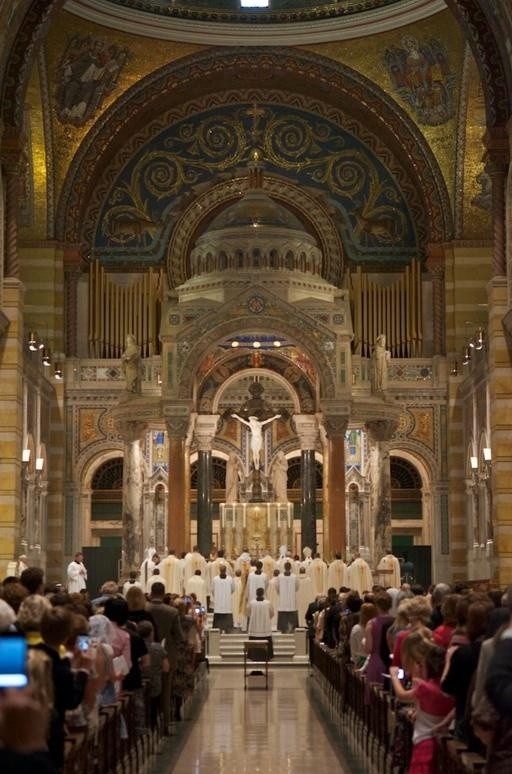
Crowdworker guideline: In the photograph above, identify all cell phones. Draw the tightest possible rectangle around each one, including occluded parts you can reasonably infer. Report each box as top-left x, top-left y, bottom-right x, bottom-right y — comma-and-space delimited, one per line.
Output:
77, 636, 91, 650
0, 630, 28, 689
195, 607, 200, 616
396, 668, 404, 679
201, 607, 205, 615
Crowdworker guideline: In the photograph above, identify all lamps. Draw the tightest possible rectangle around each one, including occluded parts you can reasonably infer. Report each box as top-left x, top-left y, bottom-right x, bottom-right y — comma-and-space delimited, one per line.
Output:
22, 449, 44, 483
469, 446, 491, 485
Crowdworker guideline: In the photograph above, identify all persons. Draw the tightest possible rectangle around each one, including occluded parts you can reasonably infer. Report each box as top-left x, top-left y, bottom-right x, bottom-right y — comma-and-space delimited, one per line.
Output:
367, 335, 392, 392
385, 36, 456, 126
55, 37, 126, 128
231, 414, 282, 471
120, 336, 145, 395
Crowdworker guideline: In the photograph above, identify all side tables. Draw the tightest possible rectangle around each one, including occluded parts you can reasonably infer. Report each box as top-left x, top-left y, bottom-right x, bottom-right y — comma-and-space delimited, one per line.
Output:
242, 639, 270, 690
